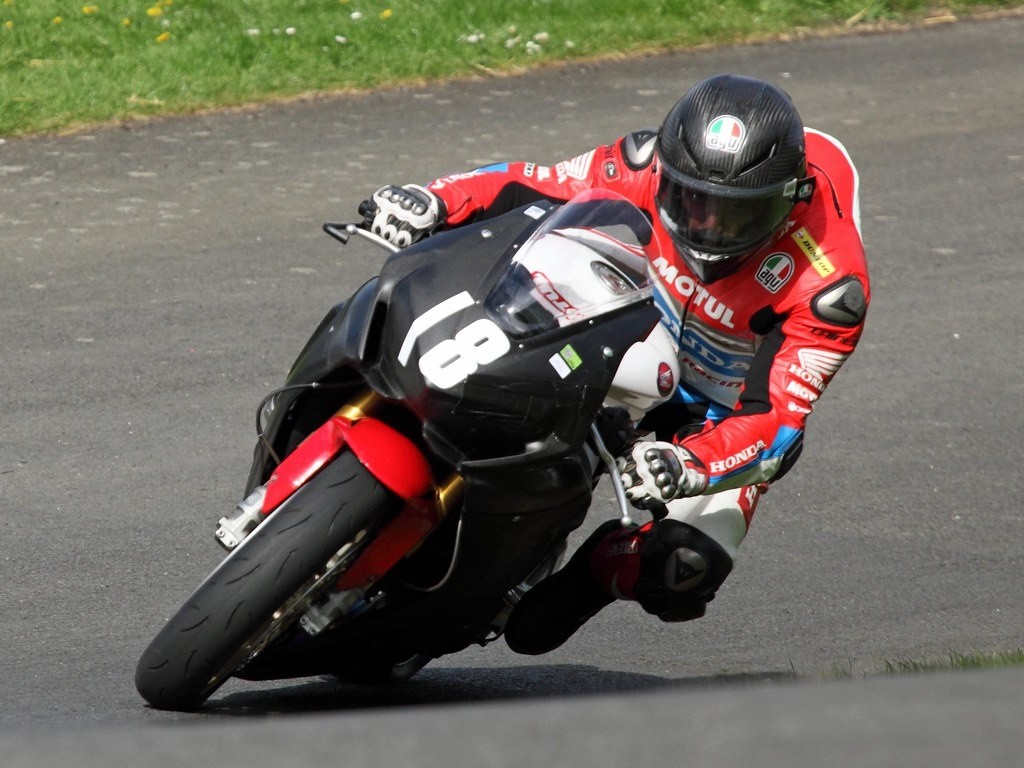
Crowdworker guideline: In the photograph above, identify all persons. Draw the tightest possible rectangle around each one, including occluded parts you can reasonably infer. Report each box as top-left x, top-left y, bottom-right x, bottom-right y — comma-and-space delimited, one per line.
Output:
366, 76, 872, 656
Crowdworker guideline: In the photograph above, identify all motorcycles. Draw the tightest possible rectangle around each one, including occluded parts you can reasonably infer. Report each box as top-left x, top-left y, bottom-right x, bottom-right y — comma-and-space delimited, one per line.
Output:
133, 187, 682, 711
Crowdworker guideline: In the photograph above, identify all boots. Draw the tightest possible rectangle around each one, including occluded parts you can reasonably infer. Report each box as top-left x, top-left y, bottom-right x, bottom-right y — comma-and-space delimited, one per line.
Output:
503, 517, 657, 656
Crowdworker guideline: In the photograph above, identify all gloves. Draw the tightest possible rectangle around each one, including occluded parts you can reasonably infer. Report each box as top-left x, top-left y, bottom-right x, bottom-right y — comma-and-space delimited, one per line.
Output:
363, 184, 447, 251
610, 441, 710, 510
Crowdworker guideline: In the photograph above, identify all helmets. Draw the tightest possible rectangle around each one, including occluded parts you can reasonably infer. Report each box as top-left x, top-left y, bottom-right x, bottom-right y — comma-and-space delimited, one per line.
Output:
655, 75, 805, 285
